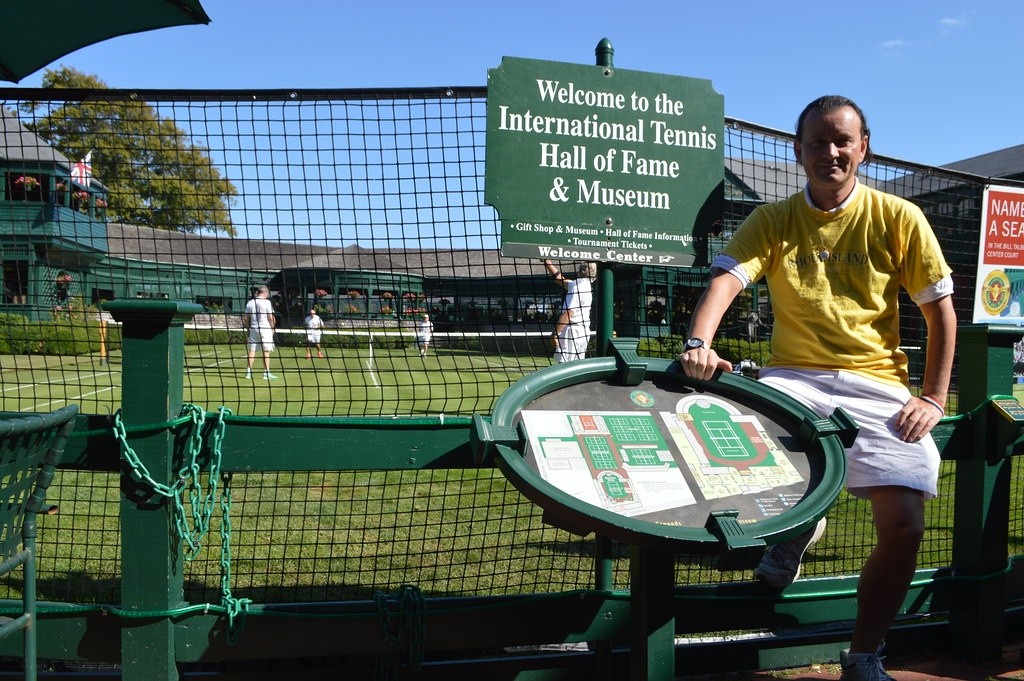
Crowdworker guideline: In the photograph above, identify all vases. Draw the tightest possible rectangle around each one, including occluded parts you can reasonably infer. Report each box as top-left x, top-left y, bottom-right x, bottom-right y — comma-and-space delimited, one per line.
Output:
384, 298, 389, 302
407, 299, 412, 303
23, 184, 32, 192
76, 199, 82, 204
96, 207, 102, 212
381, 310, 392, 314
60, 283, 67, 289
351, 295, 355, 301
318, 295, 324, 300
406, 312, 409, 314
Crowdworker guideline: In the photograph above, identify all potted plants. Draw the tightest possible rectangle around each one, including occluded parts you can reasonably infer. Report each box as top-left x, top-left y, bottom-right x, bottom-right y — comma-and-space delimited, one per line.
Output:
313, 305, 330, 317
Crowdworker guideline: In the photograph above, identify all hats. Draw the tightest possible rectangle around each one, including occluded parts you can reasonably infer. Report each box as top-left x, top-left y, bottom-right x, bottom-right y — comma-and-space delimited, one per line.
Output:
309, 309, 315, 314
423, 314, 428, 318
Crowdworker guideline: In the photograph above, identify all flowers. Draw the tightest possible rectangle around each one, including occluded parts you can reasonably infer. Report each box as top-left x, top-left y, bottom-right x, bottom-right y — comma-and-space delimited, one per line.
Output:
316, 289, 328, 296
383, 292, 393, 298
55, 184, 67, 191
405, 307, 425, 315
405, 294, 416, 300
348, 291, 360, 297
351, 305, 356, 313
378, 306, 393, 313
95, 198, 108, 207
73, 191, 89, 201
15, 177, 40, 188
54, 275, 72, 283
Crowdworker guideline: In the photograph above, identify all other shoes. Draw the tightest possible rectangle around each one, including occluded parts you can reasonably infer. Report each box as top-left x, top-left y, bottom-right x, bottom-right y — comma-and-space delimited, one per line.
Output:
306, 354, 311, 359
418, 349, 424, 357
317, 352, 323, 357
245, 372, 250, 380
262, 372, 278, 380
423, 354, 427, 358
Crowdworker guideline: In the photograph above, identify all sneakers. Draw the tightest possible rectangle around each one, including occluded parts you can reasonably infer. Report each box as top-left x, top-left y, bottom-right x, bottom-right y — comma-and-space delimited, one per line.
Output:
839, 640, 898, 681
751, 516, 827, 588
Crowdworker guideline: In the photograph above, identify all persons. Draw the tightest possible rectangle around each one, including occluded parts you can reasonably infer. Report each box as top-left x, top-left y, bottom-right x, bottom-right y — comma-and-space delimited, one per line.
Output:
304, 310, 324, 360
540, 259, 597, 364
680, 95, 957, 681
204, 299, 225, 314
243, 285, 279, 380
419, 314, 434, 357
1013, 336, 1024, 377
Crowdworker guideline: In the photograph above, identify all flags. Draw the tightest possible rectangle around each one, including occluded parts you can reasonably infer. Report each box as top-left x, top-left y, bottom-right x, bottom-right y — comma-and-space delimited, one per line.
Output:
70, 150, 92, 189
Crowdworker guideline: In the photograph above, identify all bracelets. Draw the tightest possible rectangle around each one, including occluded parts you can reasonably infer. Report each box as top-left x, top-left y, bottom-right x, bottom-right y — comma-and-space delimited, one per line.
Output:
919, 393, 945, 417
552, 331, 561, 336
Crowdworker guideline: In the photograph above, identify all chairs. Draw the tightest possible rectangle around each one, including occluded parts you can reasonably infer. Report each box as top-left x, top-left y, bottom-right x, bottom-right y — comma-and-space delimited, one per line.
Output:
0, 399, 80, 681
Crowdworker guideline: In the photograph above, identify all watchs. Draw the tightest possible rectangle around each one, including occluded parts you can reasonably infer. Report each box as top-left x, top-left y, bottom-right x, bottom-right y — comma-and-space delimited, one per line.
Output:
683, 336, 709, 352
